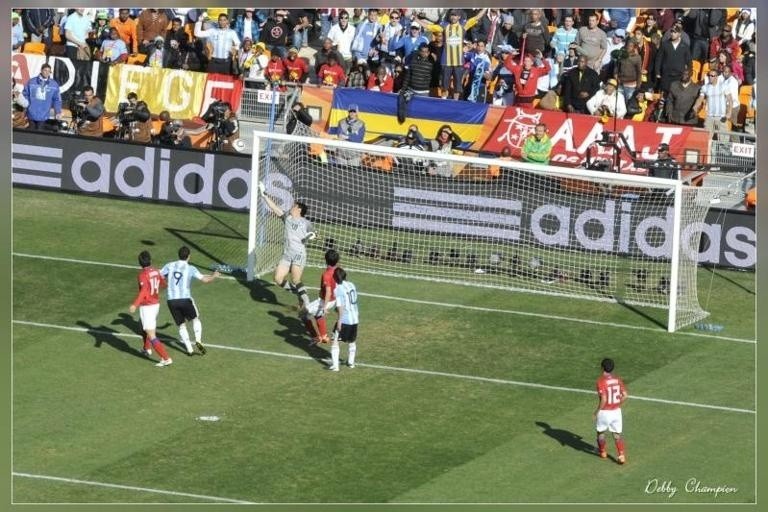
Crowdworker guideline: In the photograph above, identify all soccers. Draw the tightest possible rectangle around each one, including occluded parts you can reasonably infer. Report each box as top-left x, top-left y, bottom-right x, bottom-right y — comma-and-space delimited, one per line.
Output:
231, 139, 246, 153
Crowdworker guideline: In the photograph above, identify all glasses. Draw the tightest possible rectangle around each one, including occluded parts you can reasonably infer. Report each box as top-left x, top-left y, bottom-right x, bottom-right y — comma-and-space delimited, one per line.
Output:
707, 73, 717, 78
389, 16, 400, 21
721, 30, 730, 32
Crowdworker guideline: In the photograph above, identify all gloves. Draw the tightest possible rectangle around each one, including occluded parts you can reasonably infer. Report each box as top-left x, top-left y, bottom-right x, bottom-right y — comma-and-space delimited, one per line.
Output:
258, 181, 268, 198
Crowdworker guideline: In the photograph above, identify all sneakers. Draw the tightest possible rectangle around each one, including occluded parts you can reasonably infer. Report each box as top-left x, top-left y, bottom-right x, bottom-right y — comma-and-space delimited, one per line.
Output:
308, 336, 321, 347
153, 356, 173, 367
345, 359, 355, 368
598, 449, 607, 458
184, 350, 194, 356
321, 334, 330, 343
323, 363, 340, 372
138, 346, 152, 356
194, 340, 207, 356
615, 454, 625, 464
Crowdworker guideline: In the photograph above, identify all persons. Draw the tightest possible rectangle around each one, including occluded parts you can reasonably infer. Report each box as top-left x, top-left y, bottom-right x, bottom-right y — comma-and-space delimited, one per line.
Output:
593, 358, 626, 464
329, 267, 359, 370
159, 247, 221, 357
13, 9, 757, 177
130, 251, 173, 367
258, 182, 321, 308
306, 250, 338, 345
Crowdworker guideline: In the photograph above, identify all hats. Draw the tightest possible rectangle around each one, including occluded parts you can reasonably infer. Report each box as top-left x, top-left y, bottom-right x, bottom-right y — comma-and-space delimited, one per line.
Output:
448, 10, 461, 17
406, 130, 416, 140
347, 103, 359, 113
669, 24, 681, 34
95, 12, 109, 22
655, 143, 671, 153
255, 41, 266, 52
614, 29, 627, 41
604, 79, 618, 89
739, 9, 751, 16
410, 22, 422, 30
